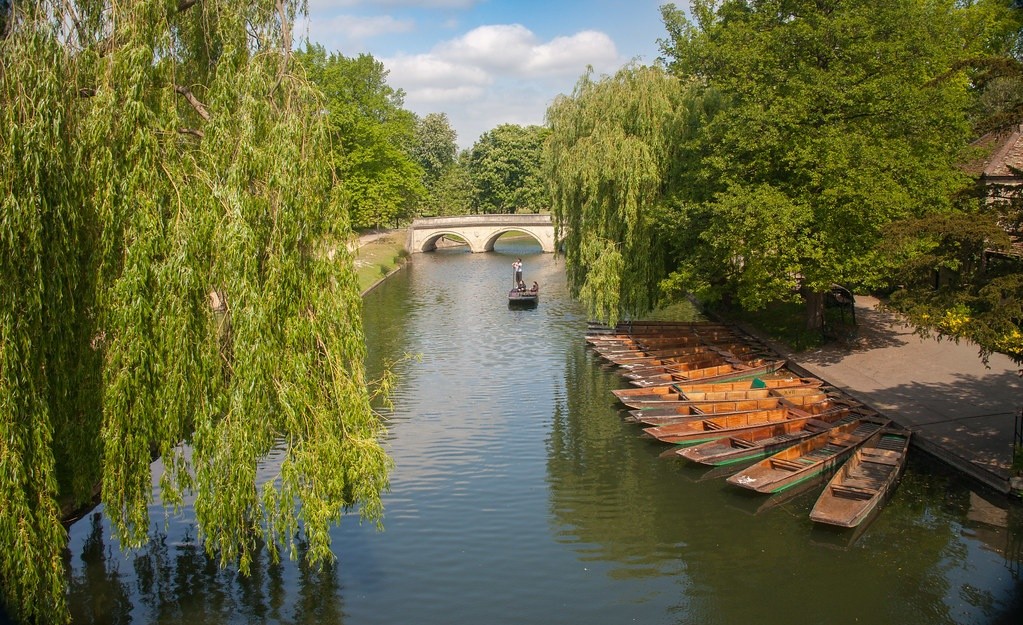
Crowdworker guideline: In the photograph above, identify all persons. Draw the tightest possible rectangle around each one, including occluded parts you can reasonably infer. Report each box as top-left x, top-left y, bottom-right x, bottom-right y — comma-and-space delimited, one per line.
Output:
512, 258, 523, 289
516, 280, 526, 292
530, 280, 539, 292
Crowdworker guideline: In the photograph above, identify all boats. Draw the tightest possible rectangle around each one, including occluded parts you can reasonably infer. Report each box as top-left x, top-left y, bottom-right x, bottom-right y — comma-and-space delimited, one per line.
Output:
724, 470, 835, 517
642, 398, 863, 446
611, 378, 824, 395
808, 455, 907, 554
675, 458, 761, 482
618, 385, 844, 411
629, 357, 787, 388
725, 416, 892, 494
508, 288, 538, 302
627, 393, 841, 427
622, 352, 778, 380
674, 408, 879, 467
808, 429, 912, 528
584, 318, 769, 370
